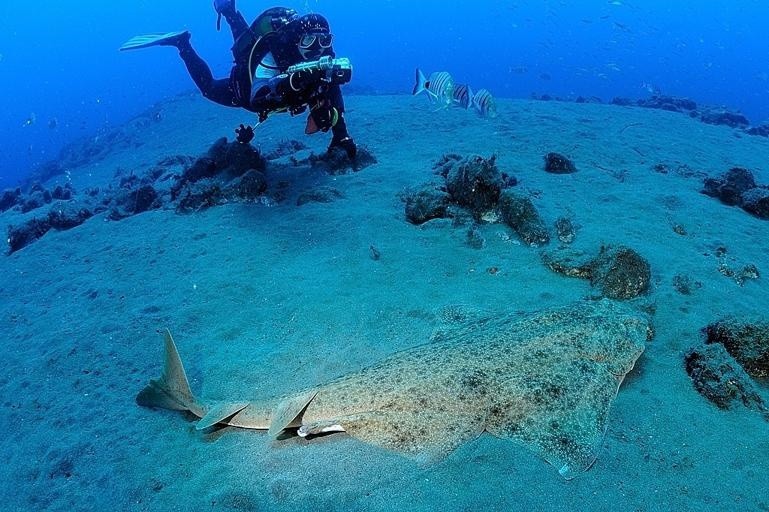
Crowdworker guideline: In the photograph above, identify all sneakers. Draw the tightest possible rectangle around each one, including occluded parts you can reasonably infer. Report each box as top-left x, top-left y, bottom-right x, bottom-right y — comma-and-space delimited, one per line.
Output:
222, 1, 236, 18
160, 32, 191, 51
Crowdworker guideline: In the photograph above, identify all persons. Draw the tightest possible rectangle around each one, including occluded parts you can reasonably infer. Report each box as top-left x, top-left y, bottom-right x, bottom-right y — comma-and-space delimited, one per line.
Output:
152, 1, 357, 174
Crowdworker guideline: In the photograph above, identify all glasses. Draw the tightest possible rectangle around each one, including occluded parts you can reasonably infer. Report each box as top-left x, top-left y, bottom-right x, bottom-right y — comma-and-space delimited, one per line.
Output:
298, 33, 333, 48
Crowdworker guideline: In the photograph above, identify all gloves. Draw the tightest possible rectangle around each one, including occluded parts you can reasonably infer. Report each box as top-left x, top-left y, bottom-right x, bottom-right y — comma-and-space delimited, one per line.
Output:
327, 133, 357, 159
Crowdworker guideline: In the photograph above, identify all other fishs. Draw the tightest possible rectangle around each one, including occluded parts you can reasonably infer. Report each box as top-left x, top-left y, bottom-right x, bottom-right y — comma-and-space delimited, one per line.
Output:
132, 290, 653, 483
479, 211, 500, 224
742, 261, 760, 279
411, 67, 497, 120
